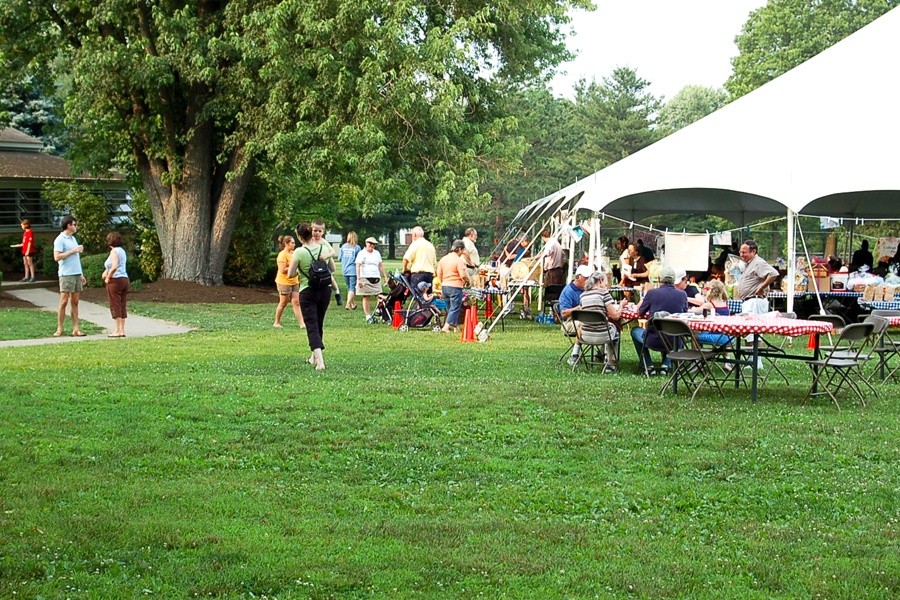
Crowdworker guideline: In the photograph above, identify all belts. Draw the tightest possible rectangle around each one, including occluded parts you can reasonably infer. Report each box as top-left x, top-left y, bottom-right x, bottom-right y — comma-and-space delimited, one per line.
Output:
742, 296, 766, 302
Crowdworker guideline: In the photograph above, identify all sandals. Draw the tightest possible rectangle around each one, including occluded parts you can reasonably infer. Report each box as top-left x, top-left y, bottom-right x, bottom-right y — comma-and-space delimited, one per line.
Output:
72, 330, 87, 335
54, 329, 64, 336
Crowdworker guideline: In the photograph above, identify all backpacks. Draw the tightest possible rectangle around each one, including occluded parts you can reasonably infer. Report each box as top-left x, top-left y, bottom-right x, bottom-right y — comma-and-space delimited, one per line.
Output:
296, 245, 332, 288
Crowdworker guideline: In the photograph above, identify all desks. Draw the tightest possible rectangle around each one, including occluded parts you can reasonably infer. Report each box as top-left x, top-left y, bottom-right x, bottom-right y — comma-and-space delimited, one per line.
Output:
469, 260, 640, 331
721, 288, 900, 386
677, 317, 835, 402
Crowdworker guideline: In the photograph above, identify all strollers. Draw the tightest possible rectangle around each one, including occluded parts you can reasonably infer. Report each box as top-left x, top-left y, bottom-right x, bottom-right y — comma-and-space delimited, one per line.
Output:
394, 273, 442, 333
364, 270, 410, 326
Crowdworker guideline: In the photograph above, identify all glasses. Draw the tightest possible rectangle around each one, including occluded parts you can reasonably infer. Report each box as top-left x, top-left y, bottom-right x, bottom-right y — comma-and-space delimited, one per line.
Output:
71, 223, 77, 226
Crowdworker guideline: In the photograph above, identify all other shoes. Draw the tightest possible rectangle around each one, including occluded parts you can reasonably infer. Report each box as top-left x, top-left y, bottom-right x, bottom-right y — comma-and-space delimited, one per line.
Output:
108, 334, 120, 340
121, 335, 126, 339
568, 349, 765, 373
336, 293, 343, 305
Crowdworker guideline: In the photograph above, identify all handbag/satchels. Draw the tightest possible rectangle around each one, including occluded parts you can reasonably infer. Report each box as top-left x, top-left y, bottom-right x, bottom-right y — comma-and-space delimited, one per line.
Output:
366, 278, 380, 284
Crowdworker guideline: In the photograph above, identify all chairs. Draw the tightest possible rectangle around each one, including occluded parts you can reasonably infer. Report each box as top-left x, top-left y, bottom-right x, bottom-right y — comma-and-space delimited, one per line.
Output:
653, 318, 728, 402
866, 342, 900, 386
824, 316, 890, 401
638, 318, 689, 378
800, 322, 876, 414
569, 309, 621, 375
553, 302, 604, 363
808, 315, 864, 375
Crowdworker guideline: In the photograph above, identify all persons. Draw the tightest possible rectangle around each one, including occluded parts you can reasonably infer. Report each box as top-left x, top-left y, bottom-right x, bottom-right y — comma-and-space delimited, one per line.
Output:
459, 227, 481, 290
51, 216, 88, 337
734, 243, 781, 375
628, 268, 690, 380
416, 281, 448, 318
437, 239, 469, 335
566, 273, 628, 374
534, 228, 566, 309
402, 226, 438, 306
309, 220, 344, 306
577, 229, 747, 320
557, 264, 620, 363
101, 232, 129, 337
495, 231, 536, 298
851, 238, 875, 276
16, 219, 37, 283
286, 223, 332, 372
337, 230, 363, 310
354, 236, 388, 322
271, 234, 306, 329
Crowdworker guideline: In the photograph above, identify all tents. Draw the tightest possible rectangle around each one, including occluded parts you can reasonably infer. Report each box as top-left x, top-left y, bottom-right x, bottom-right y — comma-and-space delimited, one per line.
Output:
462, 0, 900, 341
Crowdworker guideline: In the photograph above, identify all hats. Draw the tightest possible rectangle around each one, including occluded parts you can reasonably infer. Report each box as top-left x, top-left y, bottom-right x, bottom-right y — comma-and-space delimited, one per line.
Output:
365, 237, 378, 244
452, 240, 464, 251
576, 265, 594, 277
417, 281, 432, 291
658, 266, 676, 281
